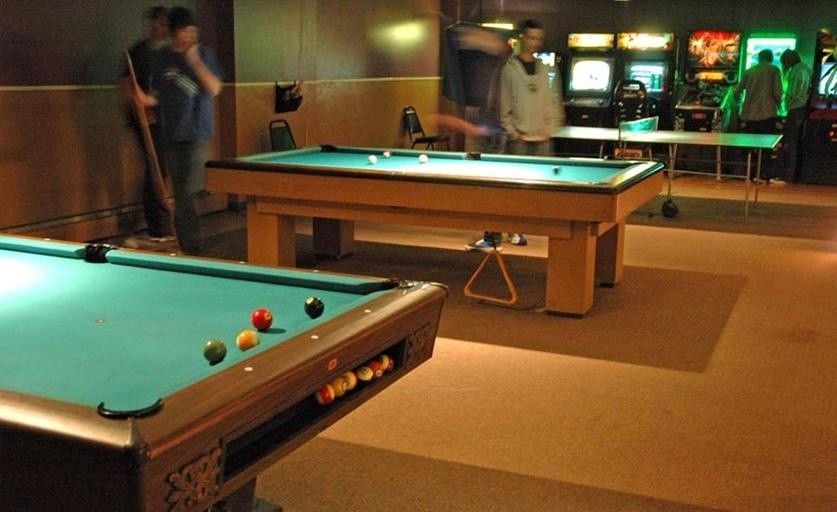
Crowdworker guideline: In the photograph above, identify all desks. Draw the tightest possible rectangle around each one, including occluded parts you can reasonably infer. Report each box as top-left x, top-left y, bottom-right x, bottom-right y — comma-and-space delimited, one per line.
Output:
494, 122, 784, 226
202, 142, 666, 318
0, 229, 453, 510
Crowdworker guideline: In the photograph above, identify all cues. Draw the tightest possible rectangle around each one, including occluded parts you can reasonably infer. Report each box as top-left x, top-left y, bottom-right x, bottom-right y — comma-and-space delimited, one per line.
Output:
123, 48, 168, 209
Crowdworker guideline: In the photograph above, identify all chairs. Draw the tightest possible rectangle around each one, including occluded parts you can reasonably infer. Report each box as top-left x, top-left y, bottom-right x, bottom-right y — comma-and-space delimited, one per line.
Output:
596, 78, 658, 162
402, 104, 450, 153
268, 118, 297, 152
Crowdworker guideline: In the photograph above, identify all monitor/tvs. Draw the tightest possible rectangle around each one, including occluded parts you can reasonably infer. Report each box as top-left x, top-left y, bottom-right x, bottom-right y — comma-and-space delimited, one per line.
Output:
815, 58, 837, 98
744, 35, 799, 78
568, 57, 614, 96
531, 51, 556, 80
621, 54, 670, 96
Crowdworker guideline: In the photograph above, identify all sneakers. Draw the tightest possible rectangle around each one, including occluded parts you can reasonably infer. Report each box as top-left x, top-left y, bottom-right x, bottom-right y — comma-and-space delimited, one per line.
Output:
508, 232, 527, 245
466, 239, 503, 252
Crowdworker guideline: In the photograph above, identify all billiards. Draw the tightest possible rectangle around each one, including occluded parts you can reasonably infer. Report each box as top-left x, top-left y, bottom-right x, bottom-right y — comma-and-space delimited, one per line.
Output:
369, 155, 376, 164
315, 354, 395, 406
252, 309, 272, 332
554, 166, 561, 172
383, 151, 390, 158
237, 330, 259, 351
204, 340, 226, 364
305, 296, 324, 318
419, 154, 428, 163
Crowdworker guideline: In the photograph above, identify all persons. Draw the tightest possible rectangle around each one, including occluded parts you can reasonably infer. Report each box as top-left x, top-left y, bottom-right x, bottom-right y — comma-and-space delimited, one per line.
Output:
132, 6, 226, 255
497, 19, 567, 158
119, 7, 177, 242
734, 50, 782, 185
769, 49, 811, 186
434, 22, 512, 253
